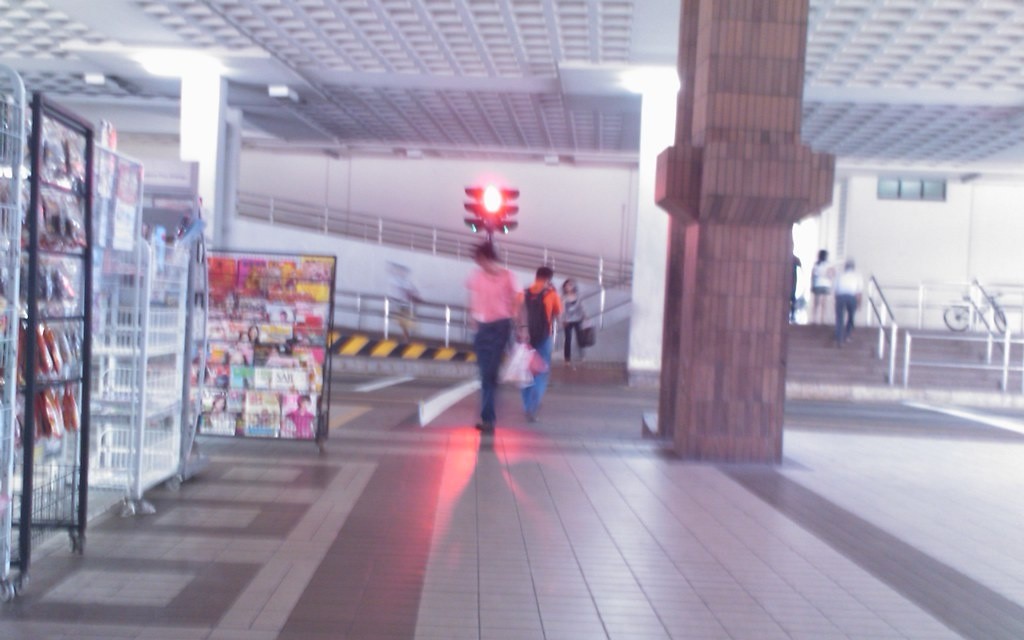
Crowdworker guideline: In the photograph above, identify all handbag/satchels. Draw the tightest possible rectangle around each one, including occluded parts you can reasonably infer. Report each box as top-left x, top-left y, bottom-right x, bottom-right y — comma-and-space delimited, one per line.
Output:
497, 335, 548, 391
577, 326, 595, 347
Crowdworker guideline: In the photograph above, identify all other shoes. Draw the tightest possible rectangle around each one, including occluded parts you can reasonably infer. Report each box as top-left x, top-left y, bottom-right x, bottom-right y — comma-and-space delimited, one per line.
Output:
476, 423, 494, 430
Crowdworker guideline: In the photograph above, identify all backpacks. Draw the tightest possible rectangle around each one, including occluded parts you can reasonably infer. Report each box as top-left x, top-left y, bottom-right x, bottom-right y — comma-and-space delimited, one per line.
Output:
523, 286, 554, 346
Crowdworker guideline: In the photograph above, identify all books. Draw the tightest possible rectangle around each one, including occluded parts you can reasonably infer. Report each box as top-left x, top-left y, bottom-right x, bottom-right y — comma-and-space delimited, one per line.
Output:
190, 257, 331, 439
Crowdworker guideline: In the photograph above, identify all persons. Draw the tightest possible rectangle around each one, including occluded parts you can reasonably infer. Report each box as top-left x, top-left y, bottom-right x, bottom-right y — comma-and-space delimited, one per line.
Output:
789, 255, 802, 322
810, 249, 835, 326
558, 279, 590, 365
281, 395, 315, 439
835, 260, 862, 347
238, 332, 250, 342
247, 325, 259, 344
512, 263, 564, 423
215, 367, 229, 389
389, 263, 424, 343
204, 394, 235, 435
280, 312, 289, 322
466, 242, 525, 432
